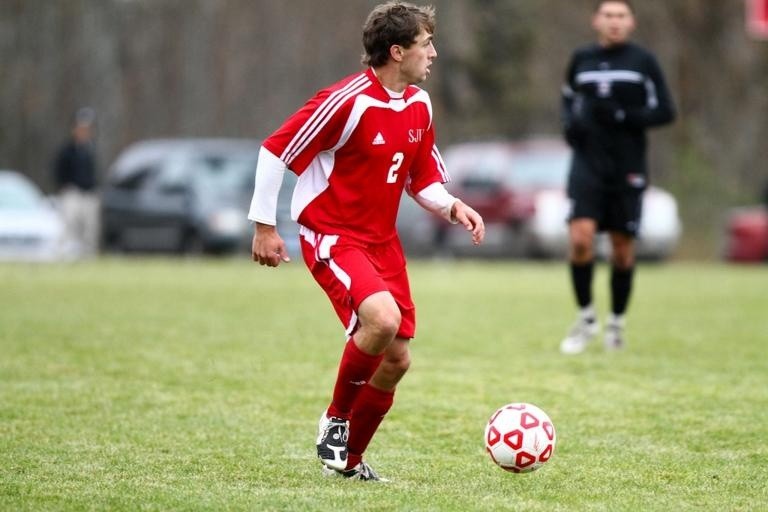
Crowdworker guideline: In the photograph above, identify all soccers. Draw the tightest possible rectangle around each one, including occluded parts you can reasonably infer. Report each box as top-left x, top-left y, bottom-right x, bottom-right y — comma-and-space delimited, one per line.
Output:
485, 403, 556, 473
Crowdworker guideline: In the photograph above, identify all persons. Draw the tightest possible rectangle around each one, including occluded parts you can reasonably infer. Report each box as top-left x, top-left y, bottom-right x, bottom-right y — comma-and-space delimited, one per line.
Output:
46, 102, 105, 263
240, 0, 486, 489
550, 1, 685, 360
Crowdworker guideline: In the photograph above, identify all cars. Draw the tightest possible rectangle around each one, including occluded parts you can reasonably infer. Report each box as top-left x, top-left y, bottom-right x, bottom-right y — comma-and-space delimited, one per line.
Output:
88, 133, 303, 259
722, 195, 768, 265
409, 135, 688, 265
0, 165, 82, 262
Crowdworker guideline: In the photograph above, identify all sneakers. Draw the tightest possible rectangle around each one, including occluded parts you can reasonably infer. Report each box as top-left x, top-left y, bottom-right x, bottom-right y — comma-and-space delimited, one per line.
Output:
322, 460, 395, 483
316, 404, 350, 472
561, 312, 625, 354
